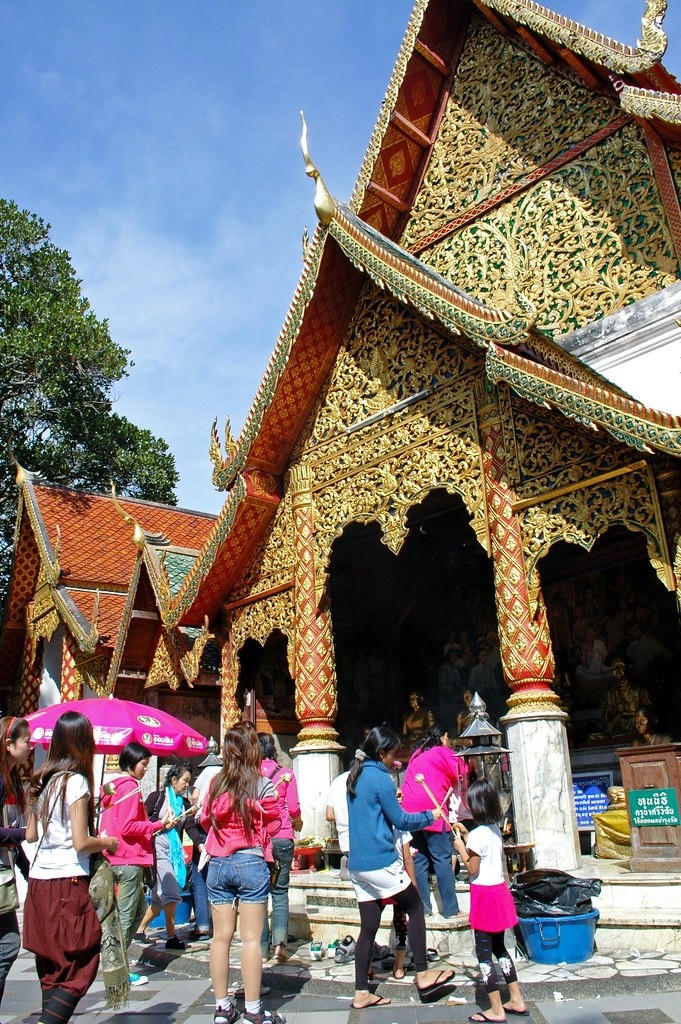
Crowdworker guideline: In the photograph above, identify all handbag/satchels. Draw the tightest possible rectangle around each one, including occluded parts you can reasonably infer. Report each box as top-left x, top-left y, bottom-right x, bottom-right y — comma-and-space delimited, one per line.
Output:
0, 869, 20, 913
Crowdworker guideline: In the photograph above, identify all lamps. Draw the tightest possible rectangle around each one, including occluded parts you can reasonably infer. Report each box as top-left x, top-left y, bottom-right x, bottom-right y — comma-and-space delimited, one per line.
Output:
450, 690, 515, 818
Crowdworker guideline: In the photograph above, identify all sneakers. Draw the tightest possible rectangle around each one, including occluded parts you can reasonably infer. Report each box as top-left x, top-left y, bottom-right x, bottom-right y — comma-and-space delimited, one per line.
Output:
333, 934, 357, 963
327, 938, 342, 959
308, 939, 326, 961
372, 941, 392, 961
128, 972, 148, 985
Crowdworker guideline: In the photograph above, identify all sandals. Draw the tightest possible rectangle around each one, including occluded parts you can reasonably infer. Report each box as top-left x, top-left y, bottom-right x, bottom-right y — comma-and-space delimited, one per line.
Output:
213, 1001, 241, 1024
243, 1001, 287, 1024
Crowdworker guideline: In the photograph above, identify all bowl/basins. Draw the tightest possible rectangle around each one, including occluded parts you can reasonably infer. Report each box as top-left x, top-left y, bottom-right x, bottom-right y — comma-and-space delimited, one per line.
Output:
519, 908, 598, 965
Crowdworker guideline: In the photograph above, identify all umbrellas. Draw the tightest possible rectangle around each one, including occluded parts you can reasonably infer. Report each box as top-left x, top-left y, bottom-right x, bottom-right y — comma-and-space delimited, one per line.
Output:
20, 692, 208, 838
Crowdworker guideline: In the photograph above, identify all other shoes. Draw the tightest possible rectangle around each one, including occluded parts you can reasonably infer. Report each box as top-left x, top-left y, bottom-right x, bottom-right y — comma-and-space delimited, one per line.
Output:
227, 980, 270, 997
189, 930, 210, 941
164, 936, 192, 950
447, 909, 470, 919
380, 952, 397, 970
134, 932, 157, 944
339, 856, 351, 880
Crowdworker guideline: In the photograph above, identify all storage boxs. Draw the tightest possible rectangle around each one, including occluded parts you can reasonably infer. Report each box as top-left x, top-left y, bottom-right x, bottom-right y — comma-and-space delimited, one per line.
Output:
291, 845, 322, 874
328, 851, 351, 869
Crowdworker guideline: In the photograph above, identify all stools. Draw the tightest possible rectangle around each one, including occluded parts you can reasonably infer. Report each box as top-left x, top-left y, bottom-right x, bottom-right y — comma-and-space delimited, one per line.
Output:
504, 841, 535, 882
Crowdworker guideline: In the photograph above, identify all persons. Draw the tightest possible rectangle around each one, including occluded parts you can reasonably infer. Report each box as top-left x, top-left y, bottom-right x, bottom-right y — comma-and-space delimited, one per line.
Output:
392, 722, 473, 919
451, 778, 530, 1024
346, 725, 455, 1009
22, 709, 120, 1024
256, 731, 303, 964
395, 685, 493, 760
326, 769, 352, 881
574, 655, 674, 747
200, 720, 287, 1024
98, 741, 225, 988
0, 716, 33, 1006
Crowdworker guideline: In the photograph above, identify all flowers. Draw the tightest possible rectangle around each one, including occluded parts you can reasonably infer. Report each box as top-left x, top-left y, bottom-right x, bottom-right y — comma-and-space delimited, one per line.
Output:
94, 782, 118, 809
280, 772, 293, 784
392, 760, 403, 770
414, 773, 425, 783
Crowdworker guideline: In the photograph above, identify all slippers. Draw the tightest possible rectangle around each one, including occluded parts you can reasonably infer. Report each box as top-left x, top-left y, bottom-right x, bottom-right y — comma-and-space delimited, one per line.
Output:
349, 994, 392, 1008
419, 969, 456, 996
392, 967, 406, 979
502, 1003, 530, 1016
427, 947, 441, 962
274, 949, 289, 958
468, 1012, 508, 1024
